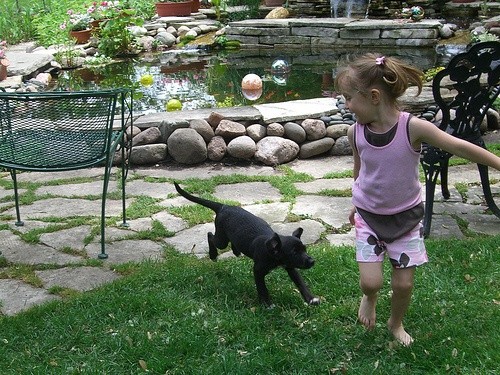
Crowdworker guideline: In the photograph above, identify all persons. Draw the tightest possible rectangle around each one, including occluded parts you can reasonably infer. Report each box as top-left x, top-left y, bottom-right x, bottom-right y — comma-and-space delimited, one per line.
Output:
337, 54, 500, 347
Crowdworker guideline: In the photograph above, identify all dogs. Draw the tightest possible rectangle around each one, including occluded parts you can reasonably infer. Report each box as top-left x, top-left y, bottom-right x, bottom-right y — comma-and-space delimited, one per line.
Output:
173, 180, 319, 309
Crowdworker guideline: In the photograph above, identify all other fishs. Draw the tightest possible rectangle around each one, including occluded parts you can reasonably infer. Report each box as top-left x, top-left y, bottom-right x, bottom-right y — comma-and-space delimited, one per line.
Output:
207, 89, 237, 99
264, 88, 301, 100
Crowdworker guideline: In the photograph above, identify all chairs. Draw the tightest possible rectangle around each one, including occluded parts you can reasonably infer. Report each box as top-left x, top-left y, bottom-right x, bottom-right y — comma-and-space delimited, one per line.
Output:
417, 41, 500, 239
0, 86, 133, 260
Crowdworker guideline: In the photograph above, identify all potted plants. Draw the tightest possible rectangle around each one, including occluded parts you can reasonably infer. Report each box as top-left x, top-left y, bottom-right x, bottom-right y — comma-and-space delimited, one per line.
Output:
155, 0, 200, 17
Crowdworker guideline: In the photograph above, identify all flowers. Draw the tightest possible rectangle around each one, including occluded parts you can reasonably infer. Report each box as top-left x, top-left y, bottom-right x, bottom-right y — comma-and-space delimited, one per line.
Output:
87, 1, 121, 20
60, 10, 94, 31
0, 39, 8, 59
162, 69, 207, 87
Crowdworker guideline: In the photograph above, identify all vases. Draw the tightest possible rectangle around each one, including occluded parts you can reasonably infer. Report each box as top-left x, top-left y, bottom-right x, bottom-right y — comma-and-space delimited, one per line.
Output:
0, 59, 10, 81
70, 29, 92, 44
159, 59, 208, 73
91, 20, 104, 27
